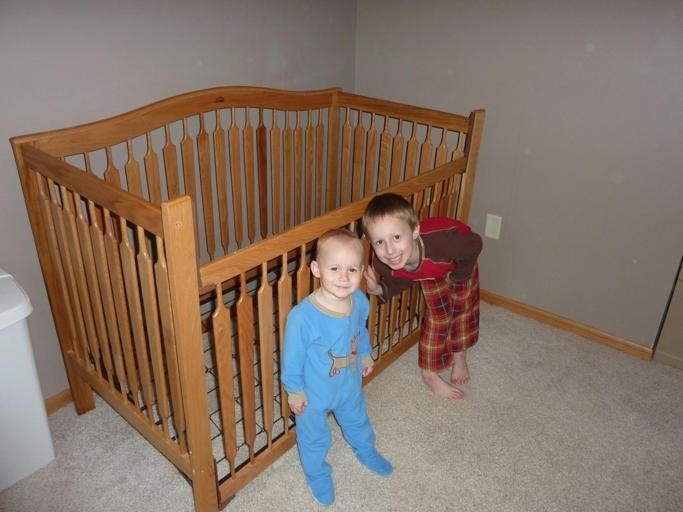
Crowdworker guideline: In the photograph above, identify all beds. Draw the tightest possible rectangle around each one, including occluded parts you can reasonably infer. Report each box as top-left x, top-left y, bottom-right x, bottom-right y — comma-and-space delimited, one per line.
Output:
9, 88, 486, 512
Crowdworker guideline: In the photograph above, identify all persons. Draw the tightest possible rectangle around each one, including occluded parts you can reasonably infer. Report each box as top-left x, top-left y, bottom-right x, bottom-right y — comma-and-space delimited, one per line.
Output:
281, 228, 394, 506
363, 192, 483, 400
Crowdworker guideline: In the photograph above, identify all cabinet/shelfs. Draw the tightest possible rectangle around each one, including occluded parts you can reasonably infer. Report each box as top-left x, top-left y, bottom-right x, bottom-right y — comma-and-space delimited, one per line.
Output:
0, 269, 55, 492
